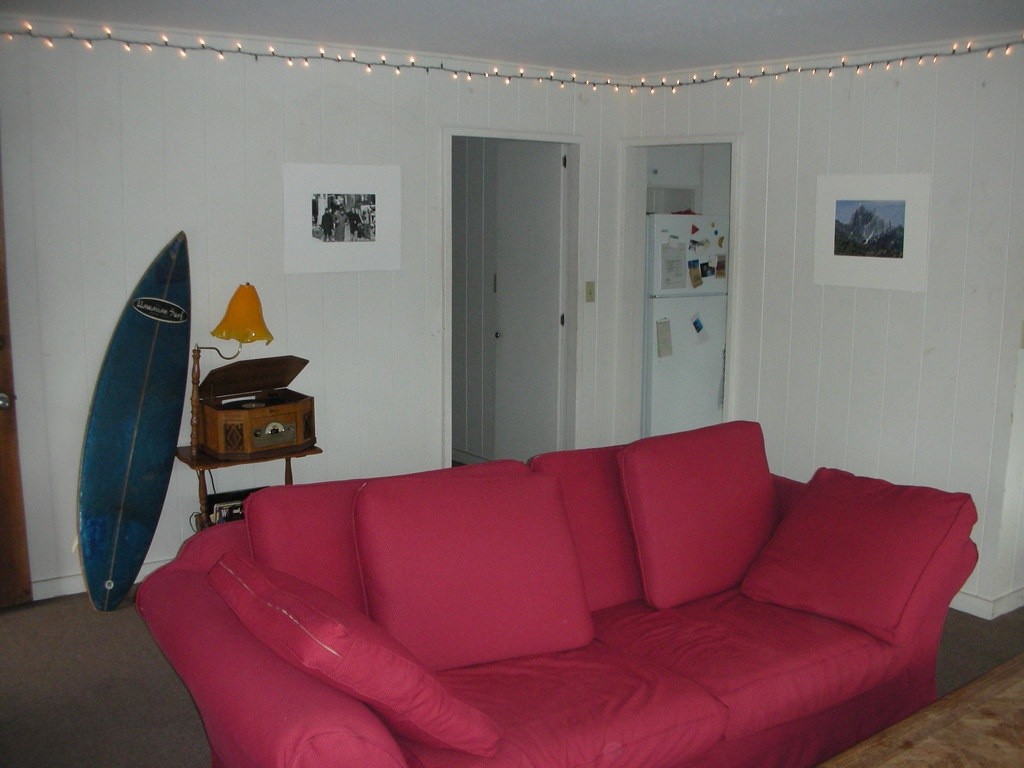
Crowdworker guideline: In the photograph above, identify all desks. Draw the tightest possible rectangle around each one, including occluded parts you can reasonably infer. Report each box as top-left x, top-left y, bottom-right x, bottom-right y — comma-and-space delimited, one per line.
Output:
171, 444, 323, 533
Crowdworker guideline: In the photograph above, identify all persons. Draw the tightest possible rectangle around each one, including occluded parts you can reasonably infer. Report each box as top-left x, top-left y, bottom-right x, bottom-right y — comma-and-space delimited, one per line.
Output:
322, 208, 361, 242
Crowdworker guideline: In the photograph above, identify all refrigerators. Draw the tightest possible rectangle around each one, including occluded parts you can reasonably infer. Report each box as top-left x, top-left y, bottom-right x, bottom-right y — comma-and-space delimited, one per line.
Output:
641, 213, 729, 438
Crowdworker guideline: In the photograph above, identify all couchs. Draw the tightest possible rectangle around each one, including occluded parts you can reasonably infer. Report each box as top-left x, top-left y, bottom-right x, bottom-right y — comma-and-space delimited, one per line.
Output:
135, 441, 981, 768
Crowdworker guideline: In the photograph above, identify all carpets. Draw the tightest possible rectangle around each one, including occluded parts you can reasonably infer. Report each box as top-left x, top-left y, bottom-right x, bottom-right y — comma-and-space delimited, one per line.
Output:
812, 653, 1024, 768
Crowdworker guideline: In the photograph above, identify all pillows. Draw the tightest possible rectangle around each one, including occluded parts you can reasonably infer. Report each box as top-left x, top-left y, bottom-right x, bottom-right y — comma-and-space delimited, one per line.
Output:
738, 464, 978, 646
208, 550, 505, 757
618, 421, 781, 608
349, 468, 597, 670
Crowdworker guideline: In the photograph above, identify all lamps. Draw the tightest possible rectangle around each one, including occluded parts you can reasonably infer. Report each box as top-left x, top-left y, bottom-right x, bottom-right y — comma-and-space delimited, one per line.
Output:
189, 281, 275, 458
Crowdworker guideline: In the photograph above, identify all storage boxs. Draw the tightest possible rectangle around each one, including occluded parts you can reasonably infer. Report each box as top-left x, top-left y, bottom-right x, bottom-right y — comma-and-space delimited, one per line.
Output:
199, 355, 318, 458
